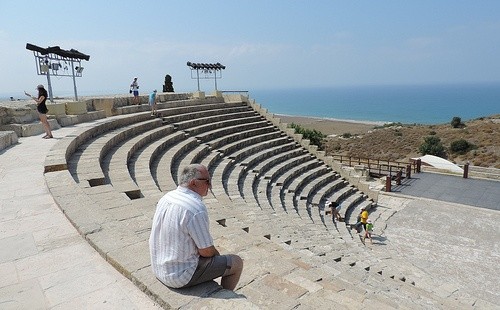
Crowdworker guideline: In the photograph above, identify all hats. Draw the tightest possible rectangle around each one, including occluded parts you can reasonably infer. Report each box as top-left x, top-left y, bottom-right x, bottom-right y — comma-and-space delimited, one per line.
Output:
366, 219, 372, 224
37, 84, 44, 90
153, 89, 157, 92
360, 206, 366, 210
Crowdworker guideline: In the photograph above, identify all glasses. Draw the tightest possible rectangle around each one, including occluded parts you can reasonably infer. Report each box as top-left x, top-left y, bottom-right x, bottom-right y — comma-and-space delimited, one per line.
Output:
197, 178, 209, 183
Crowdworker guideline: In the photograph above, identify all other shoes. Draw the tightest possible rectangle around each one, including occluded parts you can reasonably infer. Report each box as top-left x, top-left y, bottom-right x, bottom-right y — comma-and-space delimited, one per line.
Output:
151, 114, 155, 117
42, 134, 53, 139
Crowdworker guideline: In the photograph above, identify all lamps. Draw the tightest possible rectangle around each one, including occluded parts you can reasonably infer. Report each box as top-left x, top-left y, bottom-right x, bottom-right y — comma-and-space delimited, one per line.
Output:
187, 61, 225, 70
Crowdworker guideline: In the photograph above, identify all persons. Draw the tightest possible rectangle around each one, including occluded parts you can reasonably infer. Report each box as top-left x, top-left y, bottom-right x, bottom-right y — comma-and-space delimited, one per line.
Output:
130, 78, 139, 105
391, 175, 395, 181
149, 164, 243, 292
149, 88, 159, 117
10, 97, 16, 101
324, 201, 342, 222
31, 84, 53, 139
355, 206, 373, 244
